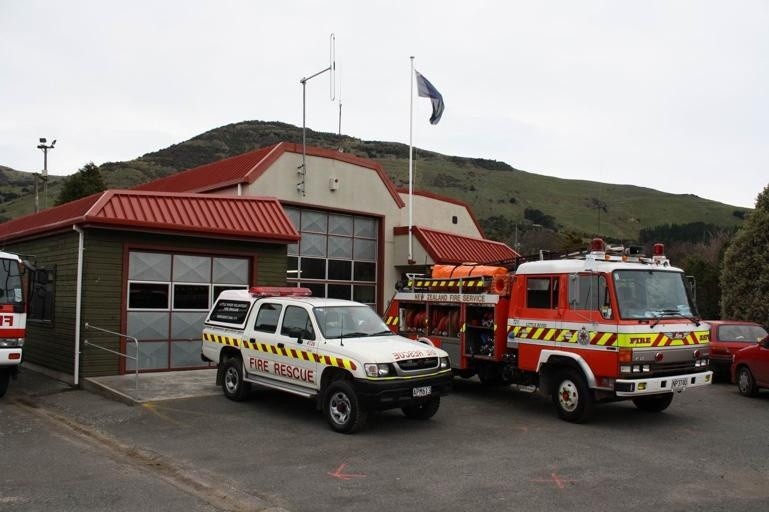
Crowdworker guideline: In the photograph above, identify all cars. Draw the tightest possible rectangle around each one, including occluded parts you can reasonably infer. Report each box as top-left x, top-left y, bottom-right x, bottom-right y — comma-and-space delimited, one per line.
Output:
698, 318, 768, 383
729, 329, 769, 396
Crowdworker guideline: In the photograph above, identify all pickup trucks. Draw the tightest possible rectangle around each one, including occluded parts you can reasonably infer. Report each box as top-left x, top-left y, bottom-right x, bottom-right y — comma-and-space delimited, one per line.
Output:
200, 289, 450, 429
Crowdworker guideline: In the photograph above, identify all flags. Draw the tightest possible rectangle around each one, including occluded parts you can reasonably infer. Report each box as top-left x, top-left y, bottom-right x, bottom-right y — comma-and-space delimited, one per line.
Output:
414, 69, 445, 126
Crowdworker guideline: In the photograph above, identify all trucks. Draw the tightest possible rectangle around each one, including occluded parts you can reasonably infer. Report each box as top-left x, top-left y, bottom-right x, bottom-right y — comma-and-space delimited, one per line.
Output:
0, 251, 39, 398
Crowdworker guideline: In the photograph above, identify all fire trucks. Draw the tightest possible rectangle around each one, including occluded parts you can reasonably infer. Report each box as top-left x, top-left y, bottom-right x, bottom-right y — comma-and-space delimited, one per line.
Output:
394, 240, 716, 423
